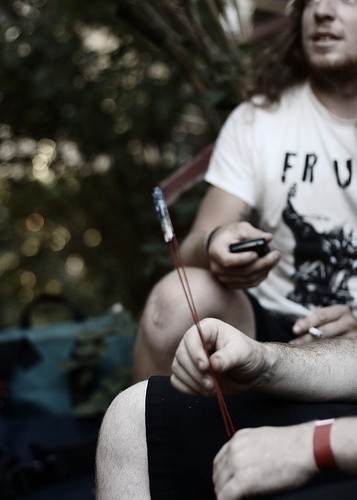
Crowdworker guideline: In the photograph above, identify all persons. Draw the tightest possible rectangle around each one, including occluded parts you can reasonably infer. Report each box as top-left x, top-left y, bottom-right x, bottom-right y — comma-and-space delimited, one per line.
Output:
132, 0, 357, 385
94, 317, 356, 500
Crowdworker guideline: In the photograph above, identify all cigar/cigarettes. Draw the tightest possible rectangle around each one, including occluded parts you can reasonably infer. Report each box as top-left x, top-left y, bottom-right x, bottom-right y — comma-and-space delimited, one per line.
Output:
308, 327, 324, 339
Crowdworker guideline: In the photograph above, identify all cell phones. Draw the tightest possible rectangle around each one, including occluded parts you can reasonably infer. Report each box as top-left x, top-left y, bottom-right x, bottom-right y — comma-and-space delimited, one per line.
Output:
230, 237, 270, 258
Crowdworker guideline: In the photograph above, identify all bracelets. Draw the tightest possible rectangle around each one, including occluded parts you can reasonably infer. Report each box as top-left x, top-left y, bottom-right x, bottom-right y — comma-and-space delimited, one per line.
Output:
204, 226, 221, 250
313, 418, 338, 474
